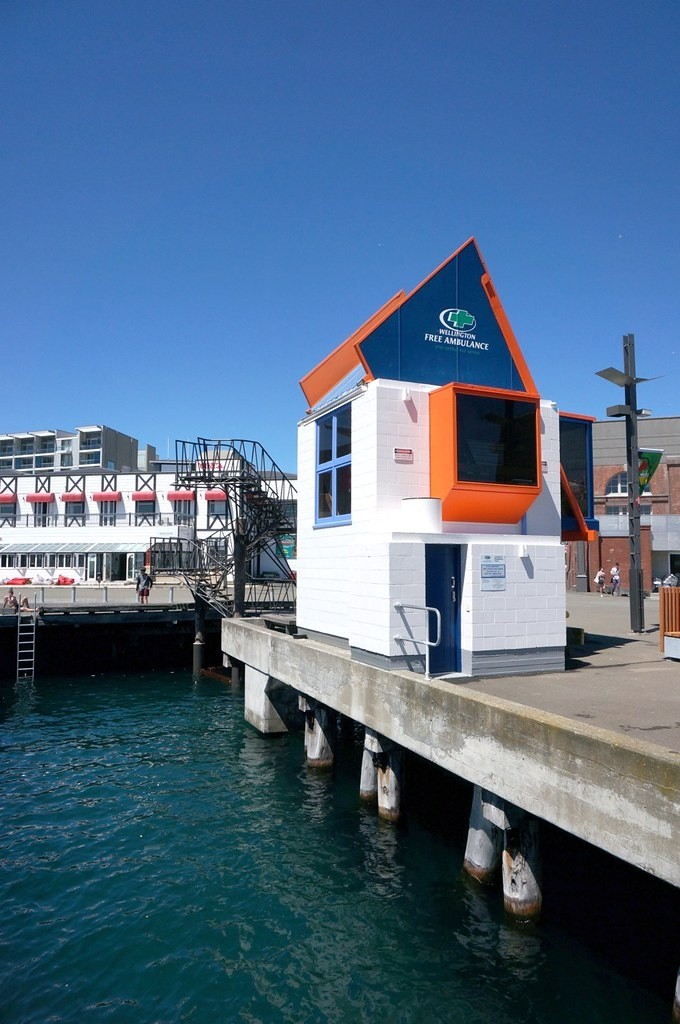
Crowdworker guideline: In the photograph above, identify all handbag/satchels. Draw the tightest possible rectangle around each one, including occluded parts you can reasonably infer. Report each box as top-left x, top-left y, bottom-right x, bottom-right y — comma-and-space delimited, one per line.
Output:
610, 577, 613, 583
599, 577, 604, 584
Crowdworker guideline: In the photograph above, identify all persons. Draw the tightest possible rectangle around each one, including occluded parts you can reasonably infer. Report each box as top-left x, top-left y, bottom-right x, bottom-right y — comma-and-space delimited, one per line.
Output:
610, 562, 620, 597
135, 566, 153, 604
597, 567, 606, 597
3, 590, 38, 611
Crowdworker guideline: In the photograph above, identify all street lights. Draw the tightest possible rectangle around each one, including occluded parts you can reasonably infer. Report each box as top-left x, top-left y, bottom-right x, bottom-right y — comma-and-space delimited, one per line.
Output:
594, 333, 666, 633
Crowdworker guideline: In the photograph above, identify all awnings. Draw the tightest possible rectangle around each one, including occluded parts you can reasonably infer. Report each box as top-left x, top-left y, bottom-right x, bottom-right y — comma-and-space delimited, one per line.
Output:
93, 491, 121, 502
205, 490, 228, 500
132, 491, 156, 501
62, 493, 85, 502
0, 494, 17, 503
26, 493, 55, 502
167, 491, 195, 500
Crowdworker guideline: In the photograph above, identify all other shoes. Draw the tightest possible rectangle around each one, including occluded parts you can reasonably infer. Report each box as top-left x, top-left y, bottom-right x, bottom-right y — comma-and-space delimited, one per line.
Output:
601, 594, 603, 597
